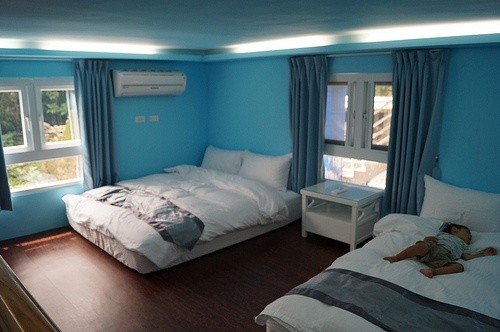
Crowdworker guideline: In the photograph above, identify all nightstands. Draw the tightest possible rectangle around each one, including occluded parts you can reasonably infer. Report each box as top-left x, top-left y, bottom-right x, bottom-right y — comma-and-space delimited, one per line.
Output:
300, 180, 383, 251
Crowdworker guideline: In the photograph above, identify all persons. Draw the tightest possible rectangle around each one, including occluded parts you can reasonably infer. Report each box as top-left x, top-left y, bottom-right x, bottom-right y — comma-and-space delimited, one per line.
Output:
383, 222, 497, 279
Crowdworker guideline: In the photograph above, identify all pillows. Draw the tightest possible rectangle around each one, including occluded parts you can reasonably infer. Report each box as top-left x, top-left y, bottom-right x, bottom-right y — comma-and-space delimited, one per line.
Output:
238, 149, 293, 192
420, 175, 500, 232
200, 145, 250, 175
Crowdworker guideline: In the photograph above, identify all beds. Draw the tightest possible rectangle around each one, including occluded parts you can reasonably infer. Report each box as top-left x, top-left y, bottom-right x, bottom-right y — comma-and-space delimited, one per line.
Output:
66, 165, 302, 274
255, 214, 500, 332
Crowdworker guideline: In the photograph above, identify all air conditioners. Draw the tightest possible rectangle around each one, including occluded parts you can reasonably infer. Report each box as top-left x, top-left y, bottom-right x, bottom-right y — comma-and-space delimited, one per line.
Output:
111, 70, 187, 98
